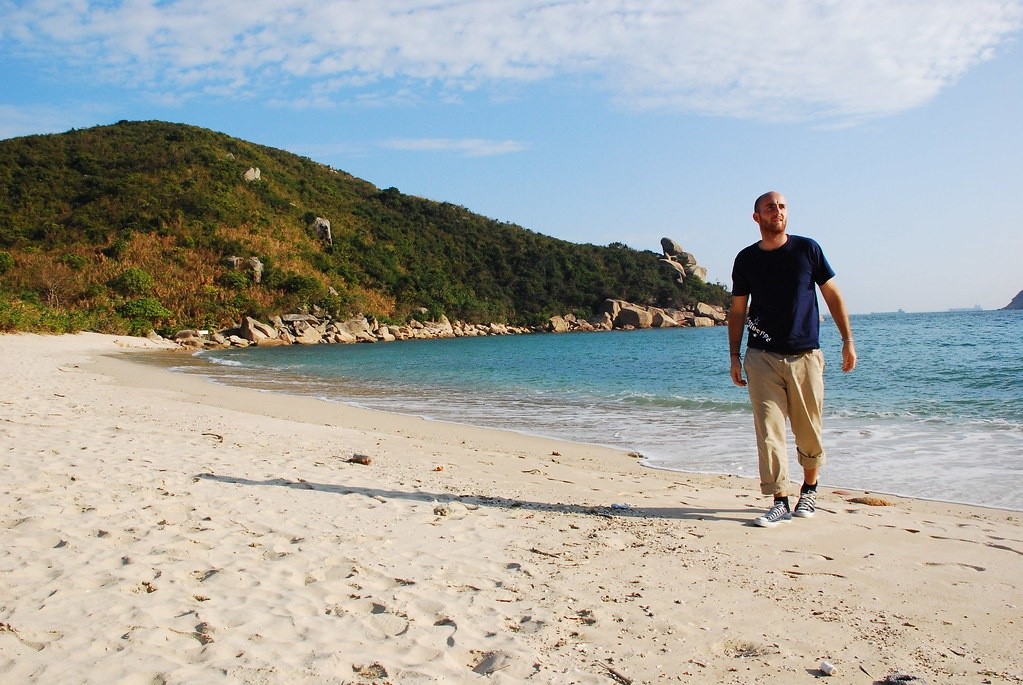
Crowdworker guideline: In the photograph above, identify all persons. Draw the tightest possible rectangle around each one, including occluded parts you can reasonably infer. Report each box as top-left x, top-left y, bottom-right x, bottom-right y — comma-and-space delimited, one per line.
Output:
727, 191, 856, 527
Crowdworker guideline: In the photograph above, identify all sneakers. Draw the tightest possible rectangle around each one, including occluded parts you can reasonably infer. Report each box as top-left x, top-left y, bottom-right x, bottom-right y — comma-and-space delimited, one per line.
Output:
754, 501, 792, 528
794, 485, 817, 517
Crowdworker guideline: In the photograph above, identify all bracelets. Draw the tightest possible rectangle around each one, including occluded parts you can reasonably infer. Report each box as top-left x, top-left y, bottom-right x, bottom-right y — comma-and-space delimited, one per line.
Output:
842, 339, 854, 342
730, 352, 742, 360
